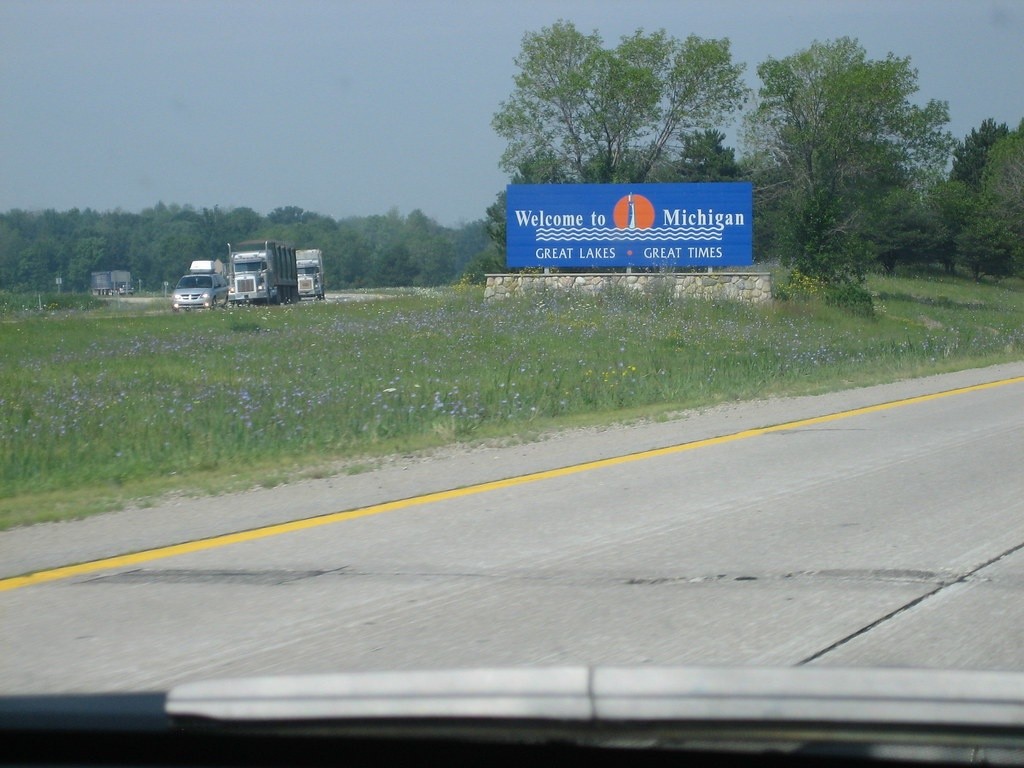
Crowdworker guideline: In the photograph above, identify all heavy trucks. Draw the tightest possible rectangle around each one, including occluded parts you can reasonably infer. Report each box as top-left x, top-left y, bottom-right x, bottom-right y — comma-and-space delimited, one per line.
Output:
90, 270, 135, 296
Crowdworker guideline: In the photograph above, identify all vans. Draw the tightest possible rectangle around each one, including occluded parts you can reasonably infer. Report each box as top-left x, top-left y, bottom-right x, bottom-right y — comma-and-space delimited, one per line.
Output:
170, 259, 227, 312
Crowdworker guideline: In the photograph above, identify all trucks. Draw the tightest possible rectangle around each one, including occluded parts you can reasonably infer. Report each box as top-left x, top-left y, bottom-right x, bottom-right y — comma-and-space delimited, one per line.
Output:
227, 239, 299, 306
295, 249, 326, 302
